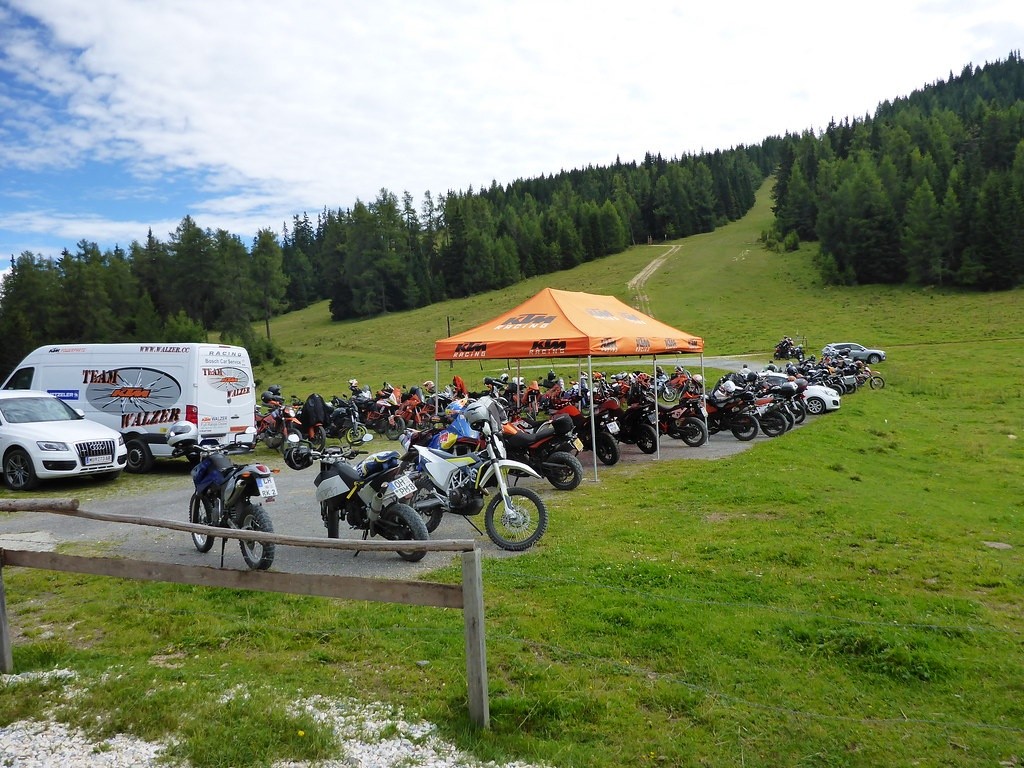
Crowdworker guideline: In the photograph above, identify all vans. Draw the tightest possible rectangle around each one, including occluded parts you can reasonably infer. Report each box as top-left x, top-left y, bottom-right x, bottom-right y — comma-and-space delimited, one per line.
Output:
0, 342, 256, 474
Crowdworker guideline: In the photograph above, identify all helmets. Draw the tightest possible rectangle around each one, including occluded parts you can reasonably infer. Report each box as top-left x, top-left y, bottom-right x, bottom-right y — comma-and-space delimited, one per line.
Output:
347, 379, 358, 389
515, 377, 524, 385
693, 374, 703, 387
548, 372, 556, 380
787, 365, 798, 375
165, 420, 198, 447
747, 372, 757, 381
500, 373, 509, 382
445, 398, 463, 418
268, 385, 281, 395
283, 447, 313, 471
261, 390, 273, 401
423, 381, 435, 392
464, 402, 491, 431
723, 381, 735, 392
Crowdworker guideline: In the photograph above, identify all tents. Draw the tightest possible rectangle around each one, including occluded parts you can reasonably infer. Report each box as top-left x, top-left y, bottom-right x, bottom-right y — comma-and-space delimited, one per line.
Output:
435, 288, 708, 482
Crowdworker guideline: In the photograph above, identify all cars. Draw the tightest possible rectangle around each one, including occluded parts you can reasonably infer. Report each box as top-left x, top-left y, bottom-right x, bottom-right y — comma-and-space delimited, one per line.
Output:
757, 370, 841, 416
0, 389, 128, 492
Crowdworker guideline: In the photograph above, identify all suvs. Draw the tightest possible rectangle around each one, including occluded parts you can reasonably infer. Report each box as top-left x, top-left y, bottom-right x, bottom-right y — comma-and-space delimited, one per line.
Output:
821, 342, 885, 365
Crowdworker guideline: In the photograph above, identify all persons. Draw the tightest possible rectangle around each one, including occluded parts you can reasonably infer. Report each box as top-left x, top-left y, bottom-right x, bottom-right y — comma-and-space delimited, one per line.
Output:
740, 364, 752, 374
764, 359, 777, 372
452, 376, 468, 402
537, 372, 559, 389
483, 373, 524, 397
804, 354, 815, 363
261, 385, 284, 415
423, 381, 437, 395
775, 335, 802, 353
714, 386, 727, 397
679, 374, 702, 404
348, 379, 372, 400
831, 348, 836, 354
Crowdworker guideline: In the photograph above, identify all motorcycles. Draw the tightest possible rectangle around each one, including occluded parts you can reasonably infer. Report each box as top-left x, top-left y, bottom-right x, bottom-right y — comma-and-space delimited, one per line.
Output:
288, 433, 431, 563
167, 426, 281, 571
253, 330, 885, 493
395, 404, 548, 551
